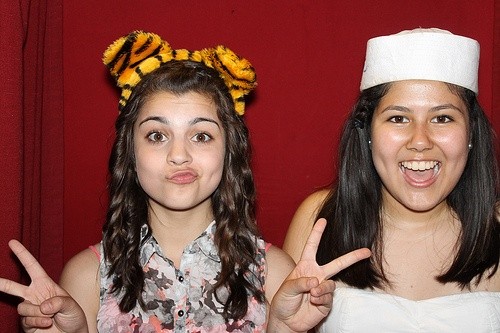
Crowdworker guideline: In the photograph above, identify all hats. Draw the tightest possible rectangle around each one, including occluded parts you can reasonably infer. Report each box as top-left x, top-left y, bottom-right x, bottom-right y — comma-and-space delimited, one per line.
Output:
360, 26, 480, 93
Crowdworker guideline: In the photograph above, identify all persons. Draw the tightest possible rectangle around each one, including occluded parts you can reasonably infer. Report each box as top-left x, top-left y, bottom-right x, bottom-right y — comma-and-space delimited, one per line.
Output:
1, 29, 372, 333
282, 27, 499, 333
